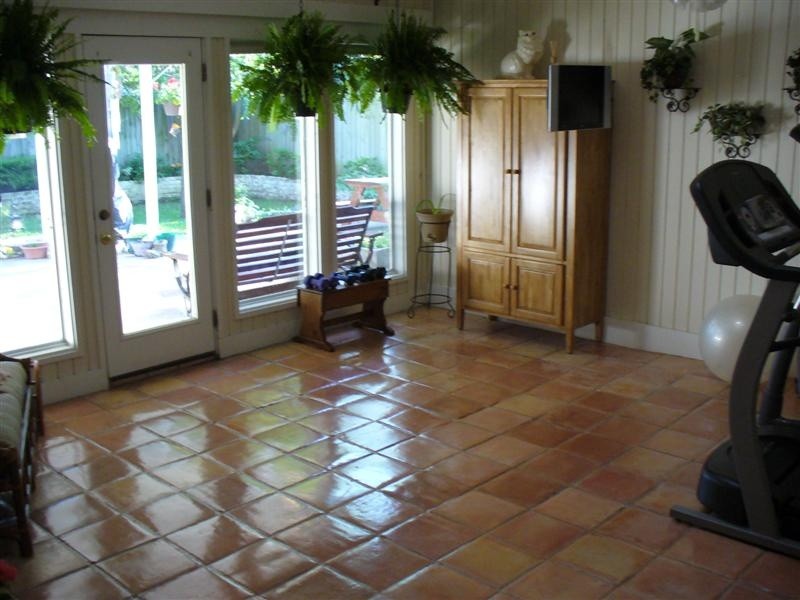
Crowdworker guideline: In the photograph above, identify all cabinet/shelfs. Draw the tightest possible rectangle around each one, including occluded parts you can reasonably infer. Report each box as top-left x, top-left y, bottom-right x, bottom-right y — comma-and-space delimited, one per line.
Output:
453, 79, 615, 355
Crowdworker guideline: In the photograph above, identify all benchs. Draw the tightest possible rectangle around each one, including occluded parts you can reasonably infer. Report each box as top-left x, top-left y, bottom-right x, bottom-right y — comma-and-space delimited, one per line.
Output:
0, 352, 45, 557
162, 206, 383, 316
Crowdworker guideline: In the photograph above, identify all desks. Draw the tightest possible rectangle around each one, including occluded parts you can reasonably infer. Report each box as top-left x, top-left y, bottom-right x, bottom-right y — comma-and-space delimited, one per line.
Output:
293, 278, 395, 352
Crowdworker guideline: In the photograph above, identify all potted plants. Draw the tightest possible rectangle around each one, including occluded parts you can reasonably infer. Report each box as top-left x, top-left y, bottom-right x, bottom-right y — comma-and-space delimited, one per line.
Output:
413, 193, 455, 242
231, 7, 484, 132
690, 101, 767, 145
640, 28, 711, 105
0, 0, 112, 160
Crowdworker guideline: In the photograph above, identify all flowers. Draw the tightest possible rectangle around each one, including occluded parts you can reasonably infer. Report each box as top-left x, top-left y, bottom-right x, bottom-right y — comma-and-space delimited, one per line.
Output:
0, 558, 20, 600
155, 84, 180, 106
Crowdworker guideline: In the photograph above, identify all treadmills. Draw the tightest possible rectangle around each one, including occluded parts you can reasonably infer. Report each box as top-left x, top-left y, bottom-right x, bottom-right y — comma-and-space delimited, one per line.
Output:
670, 158, 800, 560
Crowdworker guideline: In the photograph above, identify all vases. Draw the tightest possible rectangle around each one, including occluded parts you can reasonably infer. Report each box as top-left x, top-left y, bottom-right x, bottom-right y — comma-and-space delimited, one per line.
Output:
163, 103, 181, 116
